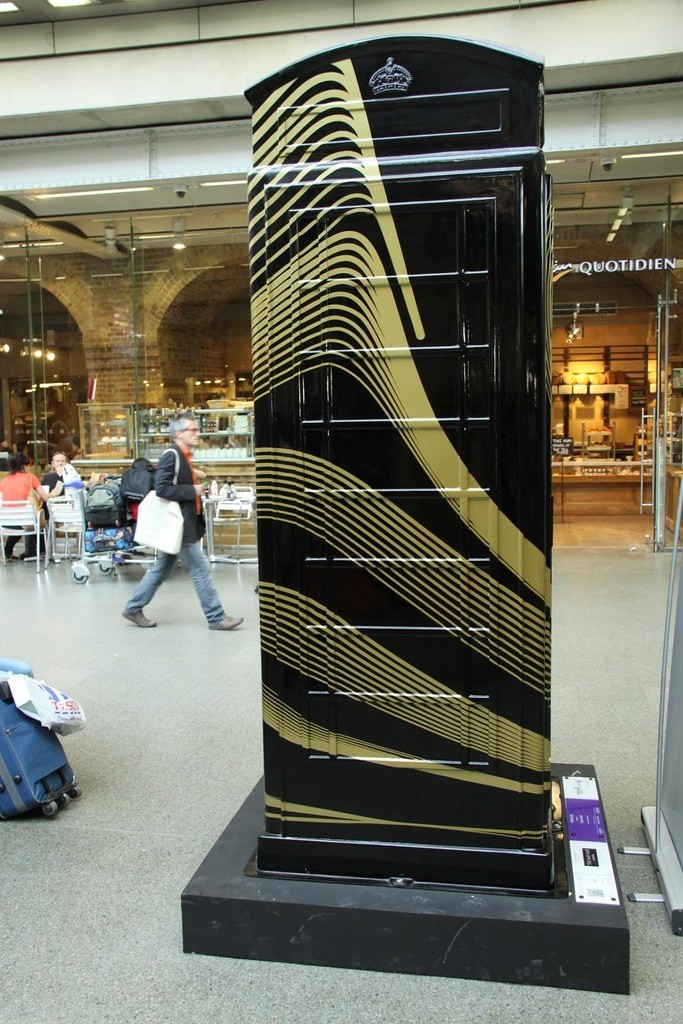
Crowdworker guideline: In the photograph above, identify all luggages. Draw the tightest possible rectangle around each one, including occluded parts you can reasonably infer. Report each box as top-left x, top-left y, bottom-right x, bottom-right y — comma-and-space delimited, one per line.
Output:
0, 675, 81, 821
84, 525, 132, 554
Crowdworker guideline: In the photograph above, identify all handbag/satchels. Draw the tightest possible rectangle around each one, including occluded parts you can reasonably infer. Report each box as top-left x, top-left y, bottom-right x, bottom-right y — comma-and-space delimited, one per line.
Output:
84, 485, 127, 528
62, 463, 82, 496
22, 473, 45, 530
121, 468, 151, 500
132, 449, 185, 555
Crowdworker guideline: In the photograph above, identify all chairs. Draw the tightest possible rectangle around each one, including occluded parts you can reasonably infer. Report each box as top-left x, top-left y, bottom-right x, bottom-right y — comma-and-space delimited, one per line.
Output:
1, 500, 50, 573
47, 495, 84, 562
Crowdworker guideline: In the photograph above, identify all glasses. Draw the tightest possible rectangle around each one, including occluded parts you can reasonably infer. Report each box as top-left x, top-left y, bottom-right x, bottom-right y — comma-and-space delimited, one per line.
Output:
178, 427, 200, 433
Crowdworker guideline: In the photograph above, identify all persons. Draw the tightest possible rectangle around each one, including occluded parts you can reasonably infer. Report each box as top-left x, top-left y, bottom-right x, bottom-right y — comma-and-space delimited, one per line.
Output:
40, 451, 75, 526
0, 454, 58, 562
121, 416, 244, 630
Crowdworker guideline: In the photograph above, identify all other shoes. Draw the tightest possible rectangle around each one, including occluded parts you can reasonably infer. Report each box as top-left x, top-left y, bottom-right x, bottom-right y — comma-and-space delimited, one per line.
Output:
1, 555, 18, 562
20, 549, 34, 560
209, 614, 243, 630
122, 610, 156, 627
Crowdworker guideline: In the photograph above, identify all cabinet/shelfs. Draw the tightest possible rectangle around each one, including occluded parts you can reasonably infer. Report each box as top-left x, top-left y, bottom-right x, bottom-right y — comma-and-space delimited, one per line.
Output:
550, 383, 683, 539
70, 401, 256, 534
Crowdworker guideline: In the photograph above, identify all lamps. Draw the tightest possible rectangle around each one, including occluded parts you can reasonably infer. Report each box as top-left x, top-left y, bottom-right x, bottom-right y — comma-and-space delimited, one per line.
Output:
620, 194, 634, 226
566, 317, 580, 345
172, 217, 186, 249
105, 226, 117, 245
